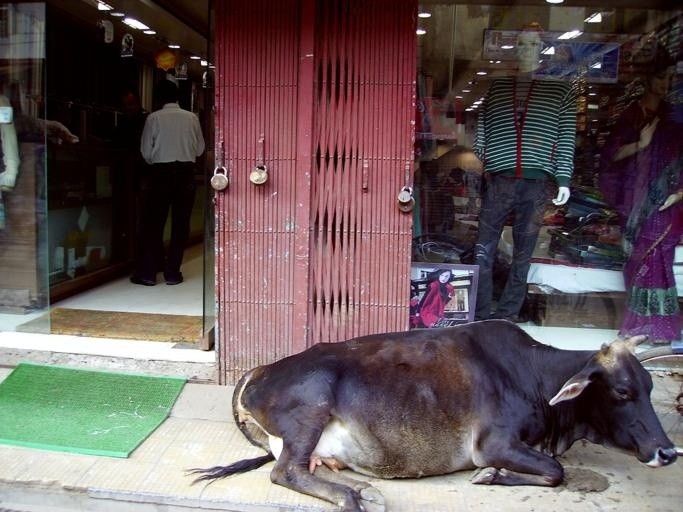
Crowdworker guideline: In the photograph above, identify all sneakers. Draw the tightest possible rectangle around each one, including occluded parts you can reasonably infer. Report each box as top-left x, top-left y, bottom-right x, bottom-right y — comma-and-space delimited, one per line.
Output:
167, 272, 182, 285
130, 273, 156, 285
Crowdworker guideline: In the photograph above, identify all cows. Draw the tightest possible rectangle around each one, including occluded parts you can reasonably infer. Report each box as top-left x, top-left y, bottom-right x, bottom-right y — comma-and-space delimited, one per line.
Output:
181, 319, 678, 512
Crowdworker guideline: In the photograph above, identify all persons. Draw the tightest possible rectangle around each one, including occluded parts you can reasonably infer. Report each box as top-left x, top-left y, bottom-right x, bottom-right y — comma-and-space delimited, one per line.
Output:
129, 79, 206, 288
472, 22, 579, 321
0, 86, 22, 236
598, 49, 683, 348
409, 269, 457, 330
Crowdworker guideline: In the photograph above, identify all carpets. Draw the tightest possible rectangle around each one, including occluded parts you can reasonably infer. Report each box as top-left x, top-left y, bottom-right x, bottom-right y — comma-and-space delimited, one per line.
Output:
0, 363, 188, 458
16, 305, 213, 343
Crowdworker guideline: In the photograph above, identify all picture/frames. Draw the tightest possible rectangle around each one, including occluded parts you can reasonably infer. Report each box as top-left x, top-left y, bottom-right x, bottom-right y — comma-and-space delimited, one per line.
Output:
410, 261, 481, 332
